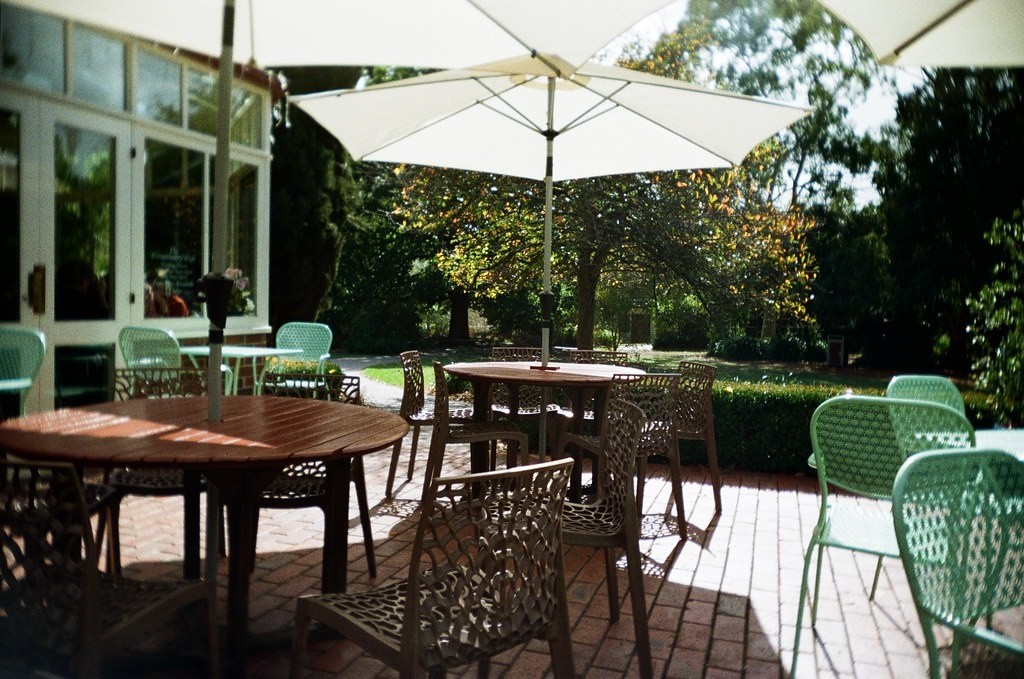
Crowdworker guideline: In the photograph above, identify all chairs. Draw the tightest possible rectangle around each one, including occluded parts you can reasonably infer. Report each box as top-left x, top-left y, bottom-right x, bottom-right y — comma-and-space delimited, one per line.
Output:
0, 319, 1022, 679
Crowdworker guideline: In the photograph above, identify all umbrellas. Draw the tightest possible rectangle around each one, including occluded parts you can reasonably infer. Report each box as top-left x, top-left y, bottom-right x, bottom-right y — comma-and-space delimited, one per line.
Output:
6, 0, 1024, 464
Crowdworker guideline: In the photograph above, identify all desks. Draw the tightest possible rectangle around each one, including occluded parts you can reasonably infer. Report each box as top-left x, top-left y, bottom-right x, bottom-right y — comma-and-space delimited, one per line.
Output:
157, 345, 304, 394
443, 360, 647, 463
0, 393, 410, 679
915, 426, 1021, 631
